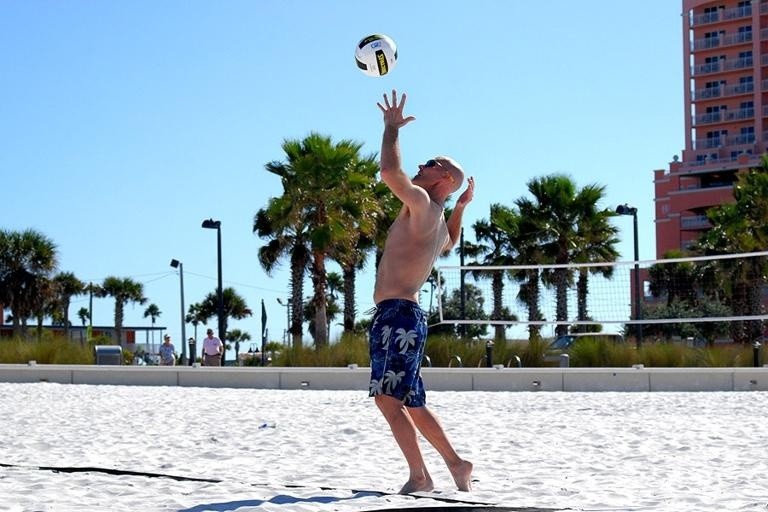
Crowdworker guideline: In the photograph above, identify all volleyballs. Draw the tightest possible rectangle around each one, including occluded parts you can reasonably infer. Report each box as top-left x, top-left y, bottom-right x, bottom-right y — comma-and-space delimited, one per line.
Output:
355, 33, 399, 77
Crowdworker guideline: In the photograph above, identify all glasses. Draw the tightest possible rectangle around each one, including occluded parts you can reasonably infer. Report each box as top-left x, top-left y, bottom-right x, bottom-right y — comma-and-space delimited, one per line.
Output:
426, 158, 457, 186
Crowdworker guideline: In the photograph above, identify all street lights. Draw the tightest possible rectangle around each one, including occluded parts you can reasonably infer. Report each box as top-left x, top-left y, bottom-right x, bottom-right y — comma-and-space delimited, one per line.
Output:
169, 257, 188, 367
275, 295, 293, 348
199, 218, 227, 367
247, 342, 260, 360
612, 200, 644, 349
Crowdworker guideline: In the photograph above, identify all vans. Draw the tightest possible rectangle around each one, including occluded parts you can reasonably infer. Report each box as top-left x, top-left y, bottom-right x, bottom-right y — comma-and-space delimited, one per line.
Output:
537, 330, 624, 368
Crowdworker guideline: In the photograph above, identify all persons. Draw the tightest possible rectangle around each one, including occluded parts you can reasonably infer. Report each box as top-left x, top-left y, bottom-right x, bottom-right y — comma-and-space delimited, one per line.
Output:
158, 334, 176, 366
132, 350, 152, 365
201, 328, 224, 366
376, 89, 474, 494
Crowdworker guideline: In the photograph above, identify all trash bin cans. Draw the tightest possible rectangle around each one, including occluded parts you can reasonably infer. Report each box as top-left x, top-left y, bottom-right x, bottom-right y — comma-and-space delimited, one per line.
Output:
93, 345, 123, 365
